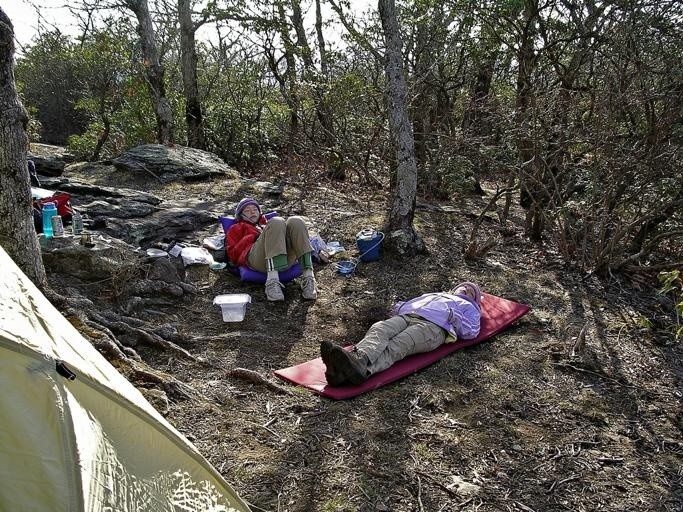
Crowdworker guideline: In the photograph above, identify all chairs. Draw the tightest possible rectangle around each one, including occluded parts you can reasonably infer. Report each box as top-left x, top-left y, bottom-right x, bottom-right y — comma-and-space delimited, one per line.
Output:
218, 211, 302, 280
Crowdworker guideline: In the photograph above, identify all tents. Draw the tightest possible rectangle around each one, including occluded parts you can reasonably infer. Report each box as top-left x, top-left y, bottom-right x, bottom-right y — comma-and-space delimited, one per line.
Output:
0, 247, 254, 512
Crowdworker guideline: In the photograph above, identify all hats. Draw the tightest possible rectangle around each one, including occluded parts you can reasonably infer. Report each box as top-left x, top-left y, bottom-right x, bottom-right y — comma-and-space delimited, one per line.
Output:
451, 281, 482, 305
234, 196, 262, 220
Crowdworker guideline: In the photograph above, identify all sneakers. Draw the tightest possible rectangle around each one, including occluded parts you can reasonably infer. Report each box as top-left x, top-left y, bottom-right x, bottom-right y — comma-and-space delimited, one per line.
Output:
264, 277, 284, 301
330, 345, 368, 387
301, 278, 317, 300
319, 339, 349, 388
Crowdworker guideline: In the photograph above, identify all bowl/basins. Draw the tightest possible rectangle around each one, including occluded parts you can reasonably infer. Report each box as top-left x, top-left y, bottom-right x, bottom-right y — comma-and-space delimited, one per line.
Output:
335, 261, 356, 274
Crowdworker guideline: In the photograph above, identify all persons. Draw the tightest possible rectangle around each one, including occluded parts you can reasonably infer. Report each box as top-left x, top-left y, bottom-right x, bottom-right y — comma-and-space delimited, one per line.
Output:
320, 280, 483, 388
224, 199, 320, 304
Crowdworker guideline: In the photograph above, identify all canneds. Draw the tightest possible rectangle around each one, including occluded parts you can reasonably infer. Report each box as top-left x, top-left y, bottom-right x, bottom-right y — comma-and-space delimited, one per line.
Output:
72, 214, 82, 234
51, 215, 63, 236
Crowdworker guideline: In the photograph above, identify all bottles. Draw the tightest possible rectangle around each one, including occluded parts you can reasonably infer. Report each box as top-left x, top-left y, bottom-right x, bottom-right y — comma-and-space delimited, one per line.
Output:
40, 202, 57, 236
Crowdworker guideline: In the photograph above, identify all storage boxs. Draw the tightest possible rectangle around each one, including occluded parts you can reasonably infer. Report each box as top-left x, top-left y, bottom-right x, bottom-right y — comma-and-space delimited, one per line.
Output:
213, 293, 251, 322
356, 229, 382, 262
